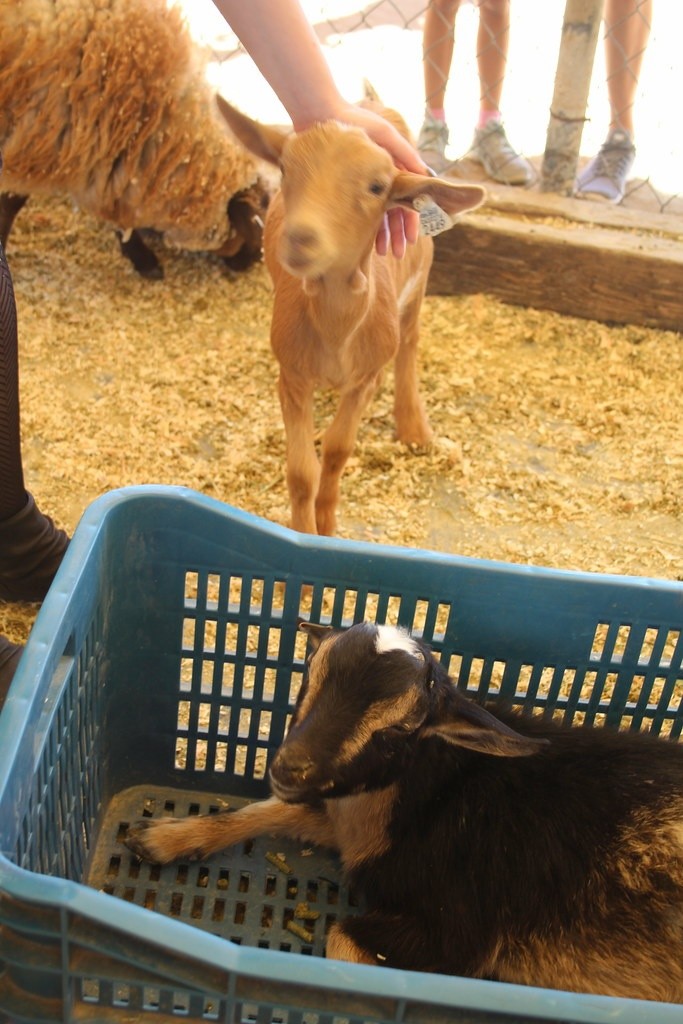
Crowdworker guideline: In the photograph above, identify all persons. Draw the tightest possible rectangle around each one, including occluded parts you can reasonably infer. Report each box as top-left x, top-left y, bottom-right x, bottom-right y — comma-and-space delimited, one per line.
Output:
419, 0, 533, 185
542, 0, 653, 204
1, 1, 436, 703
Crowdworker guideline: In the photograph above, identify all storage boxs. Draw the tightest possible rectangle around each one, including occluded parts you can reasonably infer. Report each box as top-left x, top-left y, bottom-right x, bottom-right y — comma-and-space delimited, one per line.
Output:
0, 486, 683, 1024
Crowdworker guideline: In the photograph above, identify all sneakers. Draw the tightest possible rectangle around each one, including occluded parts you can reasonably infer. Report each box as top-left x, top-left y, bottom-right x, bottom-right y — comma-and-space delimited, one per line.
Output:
573, 139, 636, 206
473, 122, 531, 182
419, 122, 449, 174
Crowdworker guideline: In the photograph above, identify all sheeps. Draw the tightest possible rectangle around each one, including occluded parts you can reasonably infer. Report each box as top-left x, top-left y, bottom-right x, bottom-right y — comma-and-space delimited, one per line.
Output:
0, 0, 257, 255
216, 92, 485, 600
121, 616, 683, 1004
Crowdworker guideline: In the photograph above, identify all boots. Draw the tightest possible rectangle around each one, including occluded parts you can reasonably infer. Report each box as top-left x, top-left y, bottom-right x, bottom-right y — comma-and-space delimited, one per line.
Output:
0, 488, 72, 601
0, 635, 24, 712
